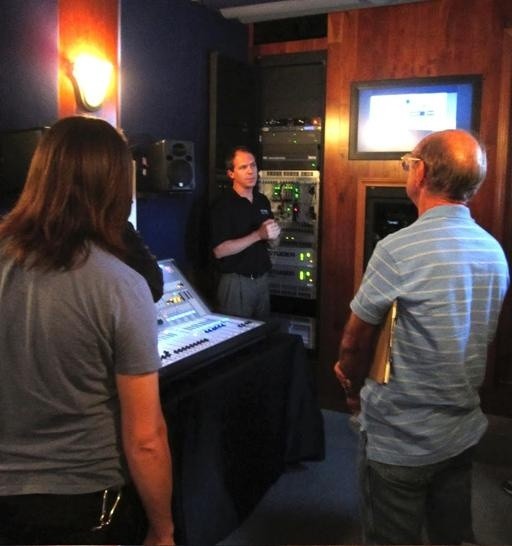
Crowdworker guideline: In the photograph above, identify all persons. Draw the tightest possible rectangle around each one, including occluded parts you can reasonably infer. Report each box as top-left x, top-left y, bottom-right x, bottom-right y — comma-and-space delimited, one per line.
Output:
1, 113, 176, 544
333, 127, 511, 544
203, 140, 285, 327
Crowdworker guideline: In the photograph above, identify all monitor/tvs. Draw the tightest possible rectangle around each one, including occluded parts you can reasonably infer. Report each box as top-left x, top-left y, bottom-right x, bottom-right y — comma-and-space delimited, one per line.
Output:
349, 82, 482, 158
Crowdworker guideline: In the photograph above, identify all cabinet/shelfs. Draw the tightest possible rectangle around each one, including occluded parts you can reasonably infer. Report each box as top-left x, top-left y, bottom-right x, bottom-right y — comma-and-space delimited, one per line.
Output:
352, 174, 419, 295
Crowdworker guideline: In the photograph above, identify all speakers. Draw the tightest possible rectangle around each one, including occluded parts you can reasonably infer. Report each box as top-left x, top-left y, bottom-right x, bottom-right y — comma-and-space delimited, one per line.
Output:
149, 136, 195, 194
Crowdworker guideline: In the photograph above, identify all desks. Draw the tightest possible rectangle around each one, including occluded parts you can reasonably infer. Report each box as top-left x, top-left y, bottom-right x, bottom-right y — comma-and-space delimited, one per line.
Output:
158, 333, 327, 545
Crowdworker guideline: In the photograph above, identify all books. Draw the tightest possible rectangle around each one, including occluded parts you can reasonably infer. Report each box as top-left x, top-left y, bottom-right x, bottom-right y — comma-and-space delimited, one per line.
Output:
363, 298, 398, 385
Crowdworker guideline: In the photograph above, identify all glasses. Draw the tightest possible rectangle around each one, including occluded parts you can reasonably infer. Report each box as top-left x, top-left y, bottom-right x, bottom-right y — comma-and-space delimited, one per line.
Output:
400, 153, 421, 171
